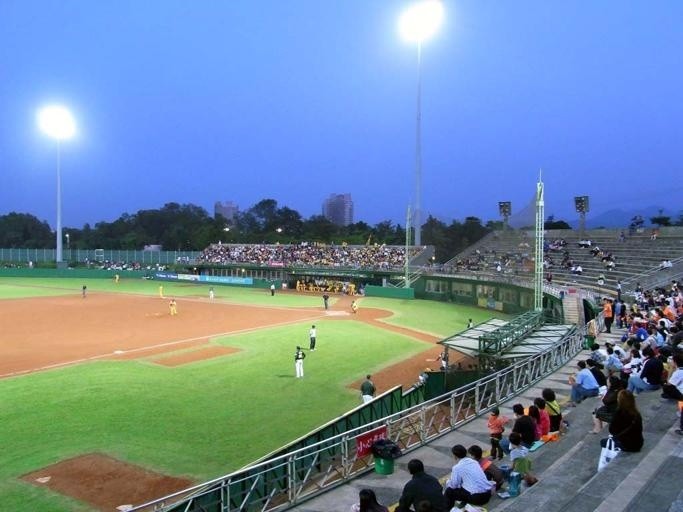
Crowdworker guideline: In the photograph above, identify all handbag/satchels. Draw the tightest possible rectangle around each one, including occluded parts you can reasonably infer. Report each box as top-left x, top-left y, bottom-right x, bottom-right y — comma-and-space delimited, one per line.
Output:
596, 438, 621, 474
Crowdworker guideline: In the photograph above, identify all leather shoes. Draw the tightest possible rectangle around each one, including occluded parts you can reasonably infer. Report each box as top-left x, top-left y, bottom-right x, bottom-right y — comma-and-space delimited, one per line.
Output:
588, 429, 600, 434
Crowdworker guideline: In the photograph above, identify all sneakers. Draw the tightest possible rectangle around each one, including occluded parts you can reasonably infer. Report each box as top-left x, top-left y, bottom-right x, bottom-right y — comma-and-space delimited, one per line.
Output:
675, 430, 683, 435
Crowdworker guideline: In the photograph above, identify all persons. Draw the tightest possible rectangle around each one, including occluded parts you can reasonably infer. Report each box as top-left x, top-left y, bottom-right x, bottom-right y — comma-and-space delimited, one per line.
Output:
3, 259, 34, 270
481, 278, 682, 484
360, 374, 375, 404
350, 300, 357, 313
80, 254, 191, 279
308, 324, 316, 351
167, 298, 176, 315
113, 272, 119, 287
351, 488, 389, 512
420, 222, 672, 285
293, 345, 305, 377
413, 498, 432, 512
80, 283, 86, 299
393, 457, 450, 511
467, 444, 505, 491
158, 283, 164, 297
442, 443, 493, 511
466, 318, 474, 329
188, 239, 420, 297
206, 287, 215, 303
424, 343, 480, 376
322, 291, 329, 309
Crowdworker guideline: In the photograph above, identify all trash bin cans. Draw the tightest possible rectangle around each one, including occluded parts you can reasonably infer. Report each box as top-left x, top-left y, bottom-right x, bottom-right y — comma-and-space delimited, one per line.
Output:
371, 438, 394, 475
582, 335, 596, 350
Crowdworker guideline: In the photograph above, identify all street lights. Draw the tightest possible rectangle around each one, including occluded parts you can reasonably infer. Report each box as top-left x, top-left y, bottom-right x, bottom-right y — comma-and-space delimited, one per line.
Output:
37, 105, 74, 262
64, 233, 70, 249
575, 195, 589, 233
497, 201, 511, 229
399, 0, 445, 245
